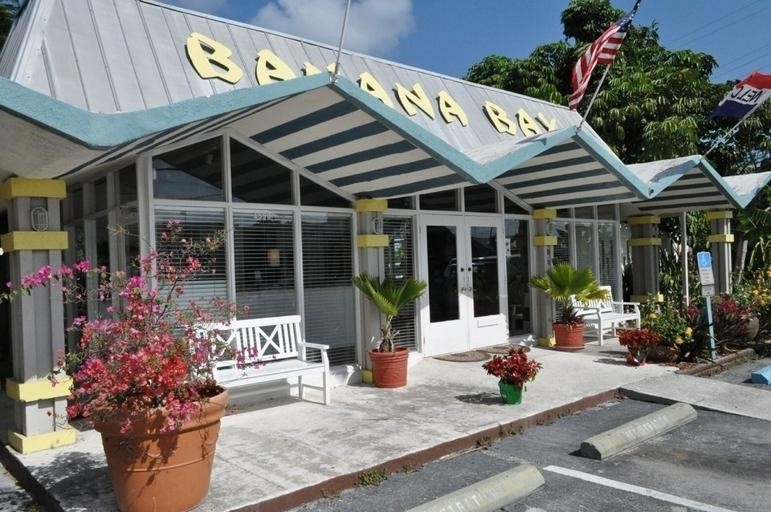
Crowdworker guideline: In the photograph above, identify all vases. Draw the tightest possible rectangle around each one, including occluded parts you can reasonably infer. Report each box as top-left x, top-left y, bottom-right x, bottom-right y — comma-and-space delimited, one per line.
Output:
630, 350, 648, 363
655, 346, 679, 363
725, 314, 760, 341
498, 377, 524, 405
87, 382, 231, 512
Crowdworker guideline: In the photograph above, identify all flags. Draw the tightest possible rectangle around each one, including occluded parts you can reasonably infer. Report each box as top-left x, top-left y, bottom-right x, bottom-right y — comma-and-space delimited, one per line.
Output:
708, 71, 770, 121
564, 0, 642, 110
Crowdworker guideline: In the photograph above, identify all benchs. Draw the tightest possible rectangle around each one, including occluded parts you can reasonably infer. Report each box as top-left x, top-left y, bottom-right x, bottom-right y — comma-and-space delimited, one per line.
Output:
510, 294, 530, 330
569, 285, 642, 347
185, 314, 333, 409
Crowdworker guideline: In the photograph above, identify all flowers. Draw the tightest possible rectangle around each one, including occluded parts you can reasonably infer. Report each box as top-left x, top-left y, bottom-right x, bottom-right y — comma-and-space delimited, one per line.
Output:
618, 328, 660, 350
481, 343, 545, 393
1, 211, 277, 436
689, 266, 771, 327
641, 292, 695, 346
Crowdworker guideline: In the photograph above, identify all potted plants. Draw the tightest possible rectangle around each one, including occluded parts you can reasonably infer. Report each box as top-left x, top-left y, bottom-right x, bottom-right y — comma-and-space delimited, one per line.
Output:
527, 259, 612, 352
351, 272, 429, 388
